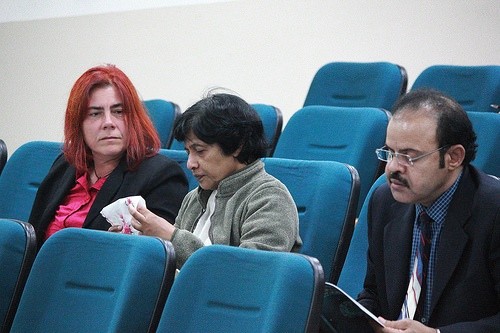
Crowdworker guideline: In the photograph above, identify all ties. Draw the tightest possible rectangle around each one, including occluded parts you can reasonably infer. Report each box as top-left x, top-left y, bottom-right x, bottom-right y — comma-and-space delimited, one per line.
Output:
397, 212, 430, 322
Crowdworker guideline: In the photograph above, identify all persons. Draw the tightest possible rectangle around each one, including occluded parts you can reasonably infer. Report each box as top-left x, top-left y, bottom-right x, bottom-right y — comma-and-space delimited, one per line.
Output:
27, 64, 188, 244
357, 90, 499, 333
129, 92, 300, 270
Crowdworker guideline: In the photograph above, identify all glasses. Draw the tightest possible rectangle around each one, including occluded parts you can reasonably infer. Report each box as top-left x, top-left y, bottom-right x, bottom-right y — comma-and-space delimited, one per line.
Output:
375, 144, 448, 165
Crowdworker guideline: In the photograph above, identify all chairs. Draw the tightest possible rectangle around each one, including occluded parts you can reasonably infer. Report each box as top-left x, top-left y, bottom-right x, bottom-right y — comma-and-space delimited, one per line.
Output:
0, 141, 66, 221
260, 158, 360, 282
0, 219, 37, 333
272, 106, 391, 208
467, 111, 500, 178
155, 245, 324, 333
303, 61, 408, 107
143, 99, 182, 149
412, 64, 500, 113
157, 150, 198, 192
171, 104, 283, 151
10, 227, 176, 333
337, 173, 389, 300
